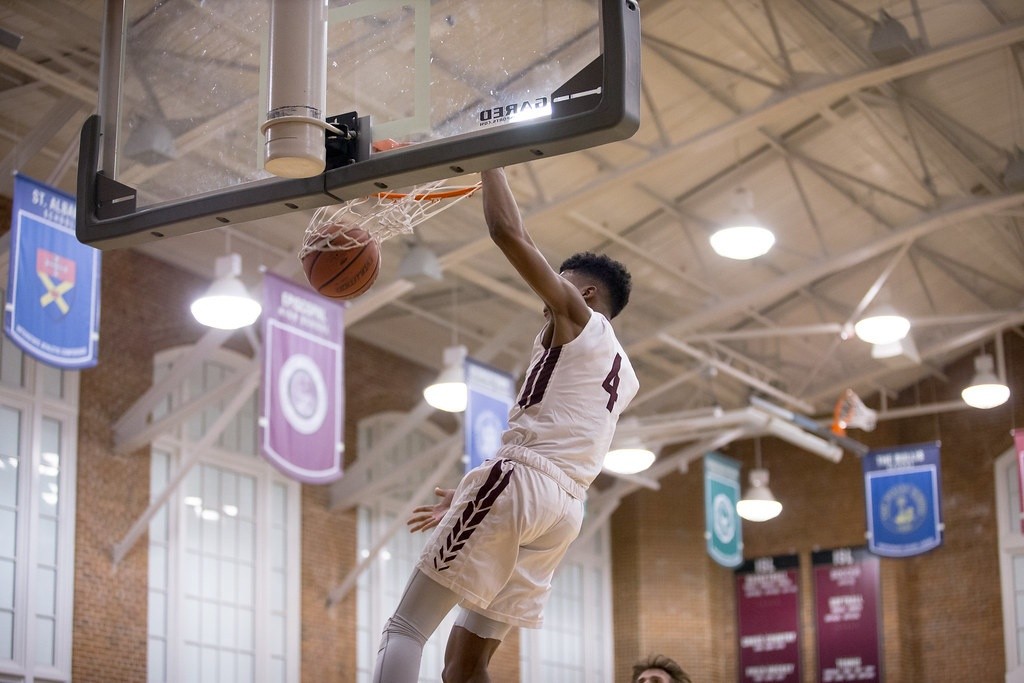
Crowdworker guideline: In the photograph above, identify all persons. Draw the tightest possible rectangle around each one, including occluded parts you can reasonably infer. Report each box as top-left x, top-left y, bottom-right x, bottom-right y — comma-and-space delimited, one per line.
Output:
633, 654, 692, 683
373, 168, 640, 683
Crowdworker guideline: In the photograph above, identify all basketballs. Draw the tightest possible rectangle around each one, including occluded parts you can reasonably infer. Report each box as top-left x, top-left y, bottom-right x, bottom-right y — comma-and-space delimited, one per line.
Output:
301, 220, 381, 302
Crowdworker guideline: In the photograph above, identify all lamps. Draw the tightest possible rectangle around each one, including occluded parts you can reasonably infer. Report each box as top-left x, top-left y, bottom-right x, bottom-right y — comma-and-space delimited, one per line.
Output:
190, 225, 261, 331
854, 282, 910, 346
121, 118, 178, 169
834, 387, 878, 434
709, 87, 777, 261
602, 415, 656, 476
961, 346, 1010, 410
736, 436, 783, 523
423, 277, 468, 414
